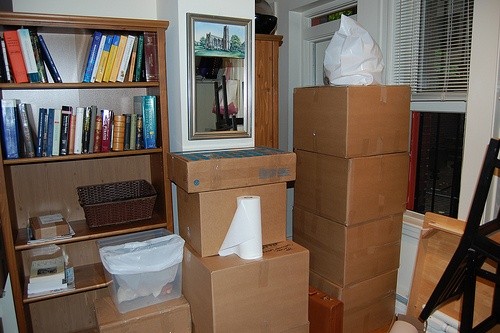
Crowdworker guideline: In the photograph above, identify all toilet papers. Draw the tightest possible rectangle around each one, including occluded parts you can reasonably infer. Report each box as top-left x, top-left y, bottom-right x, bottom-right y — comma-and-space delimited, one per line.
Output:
216, 194, 263, 262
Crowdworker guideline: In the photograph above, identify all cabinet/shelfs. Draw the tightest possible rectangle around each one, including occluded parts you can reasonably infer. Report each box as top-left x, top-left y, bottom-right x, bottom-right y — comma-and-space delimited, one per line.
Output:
0, 11, 174, 333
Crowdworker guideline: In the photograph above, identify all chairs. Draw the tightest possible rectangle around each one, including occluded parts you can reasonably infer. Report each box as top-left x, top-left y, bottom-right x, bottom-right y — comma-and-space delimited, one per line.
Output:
415, 138, 500, 333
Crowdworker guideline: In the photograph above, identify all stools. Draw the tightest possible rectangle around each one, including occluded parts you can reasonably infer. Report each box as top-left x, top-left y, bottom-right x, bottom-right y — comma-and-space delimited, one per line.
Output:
95, 293, 193, 333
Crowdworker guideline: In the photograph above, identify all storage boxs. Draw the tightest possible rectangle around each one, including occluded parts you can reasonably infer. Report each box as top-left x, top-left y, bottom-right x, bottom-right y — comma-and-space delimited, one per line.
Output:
293, 147, 408, 227
309, 268, 399, 333
293, 207, 402, 287
293, 85, 411, 158
95, 229, 185, 313
180, 240, 309, 333
167, 147, 297, 192
177, 182, 287, 257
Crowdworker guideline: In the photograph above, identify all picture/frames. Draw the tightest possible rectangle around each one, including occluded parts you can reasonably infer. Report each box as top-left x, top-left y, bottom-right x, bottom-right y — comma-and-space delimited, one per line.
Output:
186, 13, 252, 140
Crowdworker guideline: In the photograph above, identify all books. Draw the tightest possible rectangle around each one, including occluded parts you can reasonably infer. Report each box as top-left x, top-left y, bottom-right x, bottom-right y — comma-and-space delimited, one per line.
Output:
144, 32, 159, 82
83, 32, 144, 83
27, 248, 75, 298
134, 95, 158, 149
1, 99, 20, 159
0, 28, 48, 83
26, 219, 75, 245
17, 104, 143, 158
38, 35, 62, 83
